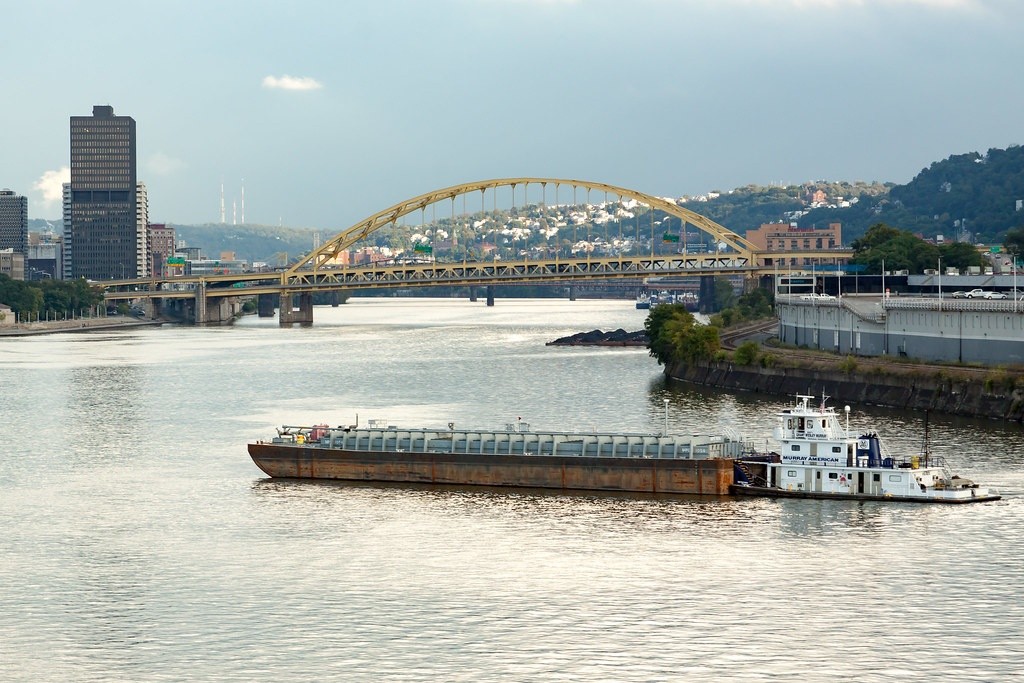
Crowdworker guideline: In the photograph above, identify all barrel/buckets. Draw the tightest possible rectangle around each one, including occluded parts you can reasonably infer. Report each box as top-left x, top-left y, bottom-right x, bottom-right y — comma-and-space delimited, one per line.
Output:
912, 456, 919, 468
297, 435, 303, 444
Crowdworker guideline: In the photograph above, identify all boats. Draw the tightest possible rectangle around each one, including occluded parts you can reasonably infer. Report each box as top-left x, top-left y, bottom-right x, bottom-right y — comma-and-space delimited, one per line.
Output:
248, 393, 1002, 508
635, 291, 700, 313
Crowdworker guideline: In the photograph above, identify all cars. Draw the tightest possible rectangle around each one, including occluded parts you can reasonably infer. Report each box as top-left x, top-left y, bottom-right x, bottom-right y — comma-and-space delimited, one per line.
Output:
799, 292, 836, 301
952, 288, 1008, 300
